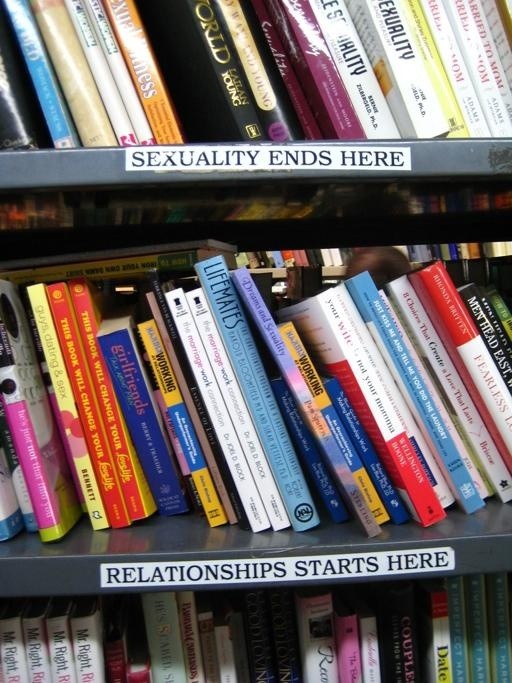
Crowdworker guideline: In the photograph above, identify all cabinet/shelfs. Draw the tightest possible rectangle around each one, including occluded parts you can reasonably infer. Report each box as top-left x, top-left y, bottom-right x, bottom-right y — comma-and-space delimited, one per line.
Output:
0, 139, 511, 592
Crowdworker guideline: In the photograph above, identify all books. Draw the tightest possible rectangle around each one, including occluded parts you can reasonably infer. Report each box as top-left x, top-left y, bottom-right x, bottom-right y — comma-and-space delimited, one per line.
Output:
1, 1, 511, 150
0, 241, 510, 545
0, 572, 511, 682
